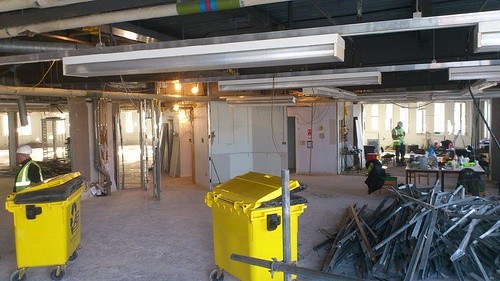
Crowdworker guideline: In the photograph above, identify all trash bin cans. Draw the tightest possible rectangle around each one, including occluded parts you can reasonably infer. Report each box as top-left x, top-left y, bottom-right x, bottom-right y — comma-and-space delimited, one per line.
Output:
204, 171, 309, 280
5, 171, 84, 280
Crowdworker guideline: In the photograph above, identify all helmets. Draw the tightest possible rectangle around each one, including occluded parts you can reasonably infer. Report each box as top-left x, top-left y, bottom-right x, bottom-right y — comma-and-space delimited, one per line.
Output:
16, 144, 32, 154
397, 122, 403, 127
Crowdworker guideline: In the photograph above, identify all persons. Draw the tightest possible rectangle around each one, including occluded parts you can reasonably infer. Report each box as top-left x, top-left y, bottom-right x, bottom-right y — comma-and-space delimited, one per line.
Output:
13, 145, 43, 193
391, 121, 407, 166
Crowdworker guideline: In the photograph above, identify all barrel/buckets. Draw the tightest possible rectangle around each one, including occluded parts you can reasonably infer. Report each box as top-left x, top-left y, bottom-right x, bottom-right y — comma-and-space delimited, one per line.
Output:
364, 138, 380, 160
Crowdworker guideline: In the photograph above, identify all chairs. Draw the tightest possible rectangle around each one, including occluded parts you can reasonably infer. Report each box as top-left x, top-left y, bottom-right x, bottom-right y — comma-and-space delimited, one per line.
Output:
373, 163, 397, 195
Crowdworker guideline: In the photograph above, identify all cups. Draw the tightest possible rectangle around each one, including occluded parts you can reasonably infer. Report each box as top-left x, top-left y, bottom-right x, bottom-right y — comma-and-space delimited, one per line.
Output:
437, 157, 443, 165
452, 160, 455, 169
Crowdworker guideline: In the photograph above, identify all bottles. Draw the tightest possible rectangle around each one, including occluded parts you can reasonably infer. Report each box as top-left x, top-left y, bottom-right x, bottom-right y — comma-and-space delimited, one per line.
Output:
453, 154, 458, 162
459, 157, 462, 168
410, 151, 414, 161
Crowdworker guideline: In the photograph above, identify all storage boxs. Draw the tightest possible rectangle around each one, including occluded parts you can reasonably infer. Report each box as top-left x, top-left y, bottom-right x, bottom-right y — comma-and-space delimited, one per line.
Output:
363, 138, 380, 163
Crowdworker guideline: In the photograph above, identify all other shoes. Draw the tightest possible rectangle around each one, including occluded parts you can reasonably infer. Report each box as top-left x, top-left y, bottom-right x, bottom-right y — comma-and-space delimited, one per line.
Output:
401, 158, 406, 162
396, 158, 399, 163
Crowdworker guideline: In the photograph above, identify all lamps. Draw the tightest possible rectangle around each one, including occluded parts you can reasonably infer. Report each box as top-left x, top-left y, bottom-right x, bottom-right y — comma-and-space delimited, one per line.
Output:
449, 66, 500, 81
472, 21, 500, 52
461, 80, 496, 97
302, 86, 339, 98
62, 33, 346, 79
333, 88, 357, 100
218, 71, 382, 93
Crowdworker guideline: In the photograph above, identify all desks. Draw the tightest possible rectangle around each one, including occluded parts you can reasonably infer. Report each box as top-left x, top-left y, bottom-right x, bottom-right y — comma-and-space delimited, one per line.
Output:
342, 150, 363, 173
405, 165, 440, 188
441, 160, 485, 191
